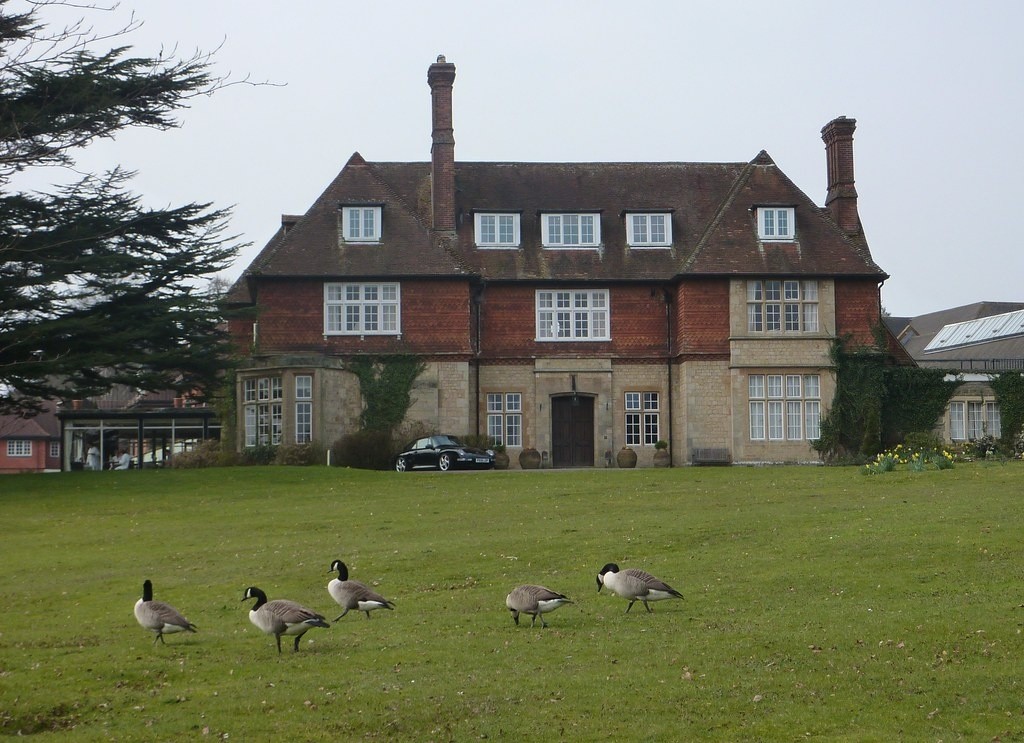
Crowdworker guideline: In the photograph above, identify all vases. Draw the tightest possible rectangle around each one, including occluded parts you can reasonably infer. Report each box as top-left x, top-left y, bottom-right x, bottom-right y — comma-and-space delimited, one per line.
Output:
518, 449, 541, 469
617, 449, 638, 469
72, 399, 84, 410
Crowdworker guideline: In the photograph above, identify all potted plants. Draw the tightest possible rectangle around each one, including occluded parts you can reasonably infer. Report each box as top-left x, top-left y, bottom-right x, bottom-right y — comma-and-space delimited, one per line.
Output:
493, 443, 510, 470
652, 441, 670, 468
173, 394, 183, 408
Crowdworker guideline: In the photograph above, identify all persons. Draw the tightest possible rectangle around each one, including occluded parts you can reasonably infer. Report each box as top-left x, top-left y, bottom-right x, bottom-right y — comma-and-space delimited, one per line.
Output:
109, 451, 121, 470
114, 448, 130, 470
86, 442, 99, 470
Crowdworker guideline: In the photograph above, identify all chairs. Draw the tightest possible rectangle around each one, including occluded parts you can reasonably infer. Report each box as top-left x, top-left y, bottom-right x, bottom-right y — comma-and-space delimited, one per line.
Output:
128, 460, 134, 470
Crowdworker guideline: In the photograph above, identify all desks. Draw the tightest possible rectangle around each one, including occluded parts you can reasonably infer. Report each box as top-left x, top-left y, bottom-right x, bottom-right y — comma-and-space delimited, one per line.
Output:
107, 461, 119, 471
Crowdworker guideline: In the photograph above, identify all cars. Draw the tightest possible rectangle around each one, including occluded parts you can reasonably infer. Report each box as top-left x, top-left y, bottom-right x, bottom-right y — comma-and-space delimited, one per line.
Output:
390, 434, 496, 471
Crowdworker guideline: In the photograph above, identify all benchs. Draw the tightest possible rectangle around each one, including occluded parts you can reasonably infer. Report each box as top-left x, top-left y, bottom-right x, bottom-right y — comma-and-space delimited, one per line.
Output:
691, 448, 733, 467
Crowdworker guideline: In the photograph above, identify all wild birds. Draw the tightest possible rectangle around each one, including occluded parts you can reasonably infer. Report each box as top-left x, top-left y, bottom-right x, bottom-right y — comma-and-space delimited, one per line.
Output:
134, 579, 199, 646
240, 586, 330, 654
505, 584, 574, 630
596, 562, 684, 613
328, 559, 397, 622
